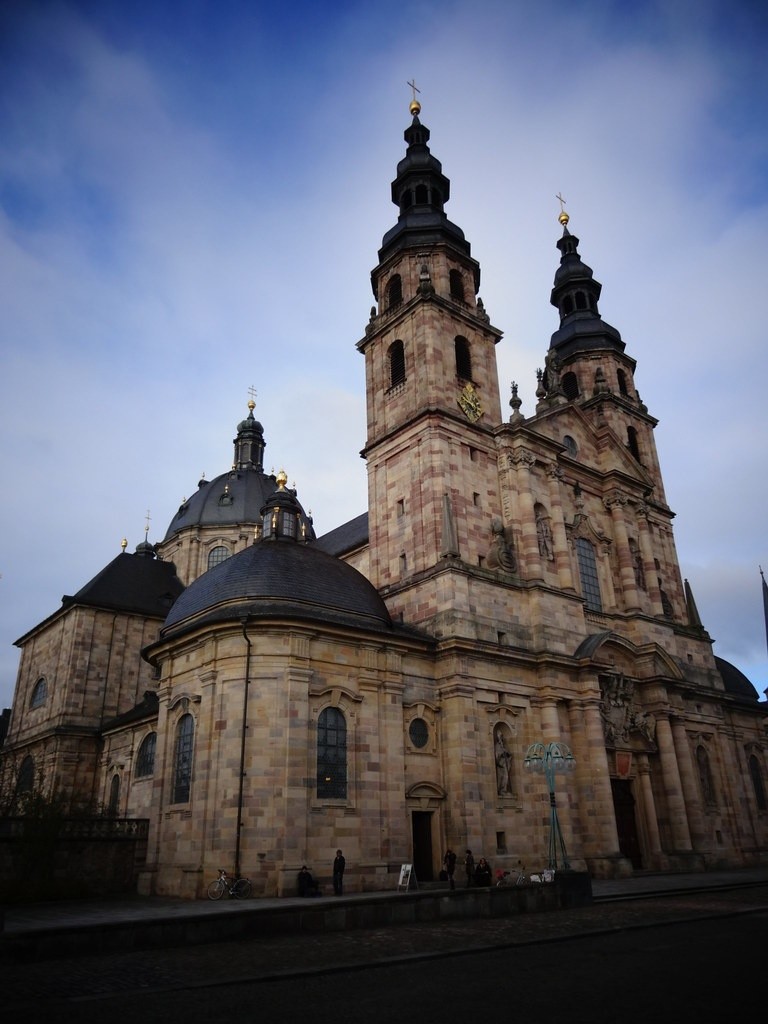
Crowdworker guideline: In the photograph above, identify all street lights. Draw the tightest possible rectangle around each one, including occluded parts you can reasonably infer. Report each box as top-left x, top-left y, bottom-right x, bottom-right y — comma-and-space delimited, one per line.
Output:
523, 741, 576, 869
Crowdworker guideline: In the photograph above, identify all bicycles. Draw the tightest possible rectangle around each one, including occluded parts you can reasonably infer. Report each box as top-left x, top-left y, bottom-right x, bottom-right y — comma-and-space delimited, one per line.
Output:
207, 869, 253, 900
497, 871, 530, 885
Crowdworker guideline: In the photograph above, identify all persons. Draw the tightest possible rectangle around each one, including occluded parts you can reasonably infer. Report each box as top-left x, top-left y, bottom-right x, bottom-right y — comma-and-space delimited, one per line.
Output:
476, 857, 492, 887
464, 849, 475, 888
333, 850, 345, 896
443, 849, 456, 893
297, 866, 321, 897
